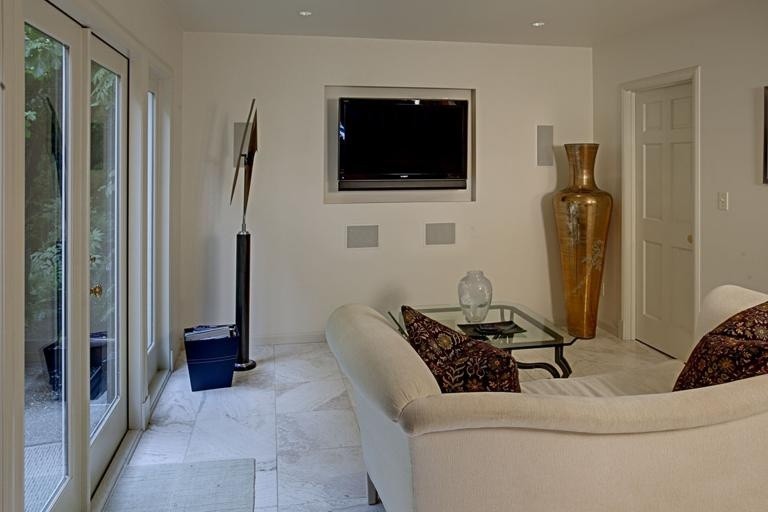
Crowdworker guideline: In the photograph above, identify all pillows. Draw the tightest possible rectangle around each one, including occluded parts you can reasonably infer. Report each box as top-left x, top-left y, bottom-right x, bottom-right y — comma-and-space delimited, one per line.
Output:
402, 305, 521, 392
672, 301, 768, 390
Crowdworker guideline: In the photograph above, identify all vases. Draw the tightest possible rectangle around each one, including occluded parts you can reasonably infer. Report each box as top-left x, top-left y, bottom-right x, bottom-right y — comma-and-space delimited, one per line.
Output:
552, 143, 613, 339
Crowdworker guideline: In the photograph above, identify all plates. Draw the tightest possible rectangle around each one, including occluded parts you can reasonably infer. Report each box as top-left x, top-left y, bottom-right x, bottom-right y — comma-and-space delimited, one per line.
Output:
457, 321, 526, 337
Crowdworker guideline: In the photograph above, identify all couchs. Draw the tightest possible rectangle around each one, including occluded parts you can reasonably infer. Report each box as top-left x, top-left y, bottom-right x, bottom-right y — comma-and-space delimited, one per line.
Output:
325, 284, 768, 512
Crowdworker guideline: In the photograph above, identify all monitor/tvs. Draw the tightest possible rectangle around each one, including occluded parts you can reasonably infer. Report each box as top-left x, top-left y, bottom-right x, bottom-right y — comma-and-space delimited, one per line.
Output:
336, 96, 469, 190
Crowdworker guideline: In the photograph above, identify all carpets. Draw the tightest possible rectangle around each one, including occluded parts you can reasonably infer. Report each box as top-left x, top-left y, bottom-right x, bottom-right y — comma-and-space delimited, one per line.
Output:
24, 441, 65, 511
102, 458, 255, 511
